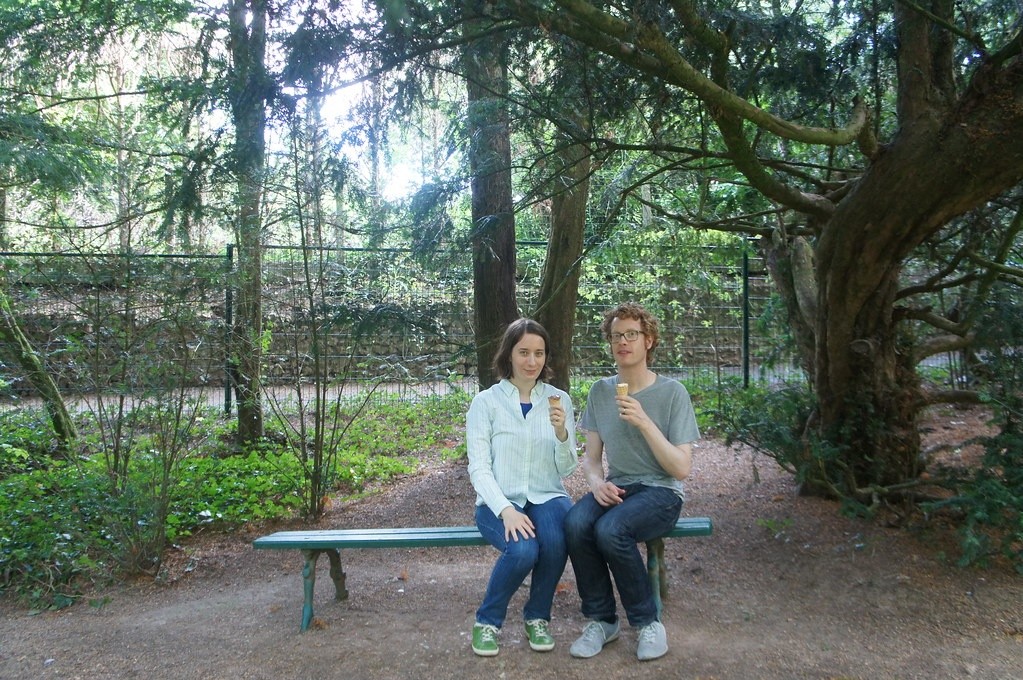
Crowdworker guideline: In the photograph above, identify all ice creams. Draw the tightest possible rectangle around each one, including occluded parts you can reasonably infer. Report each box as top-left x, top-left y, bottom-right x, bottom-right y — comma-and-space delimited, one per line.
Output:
615, 383, 628, 396
548, 395, 561, 406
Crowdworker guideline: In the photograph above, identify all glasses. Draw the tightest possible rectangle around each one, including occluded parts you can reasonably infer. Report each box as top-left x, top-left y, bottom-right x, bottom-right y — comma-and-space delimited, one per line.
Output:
607, 330, 646, 343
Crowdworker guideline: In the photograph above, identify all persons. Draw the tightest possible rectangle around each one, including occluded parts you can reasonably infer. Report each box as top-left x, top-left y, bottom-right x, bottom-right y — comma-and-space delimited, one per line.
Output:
562, 304, 700, 661
464, 317, 580, 657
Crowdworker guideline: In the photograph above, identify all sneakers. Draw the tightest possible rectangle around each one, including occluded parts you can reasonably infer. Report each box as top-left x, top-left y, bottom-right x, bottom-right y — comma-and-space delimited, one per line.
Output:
569, 615, 619, 658
524, 618, 555, 652
637, 620, 668, 660
472, 621, 499, 656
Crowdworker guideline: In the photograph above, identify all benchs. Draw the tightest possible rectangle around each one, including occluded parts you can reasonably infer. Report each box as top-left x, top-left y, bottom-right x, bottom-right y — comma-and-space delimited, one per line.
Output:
251, 516, 714, 632
